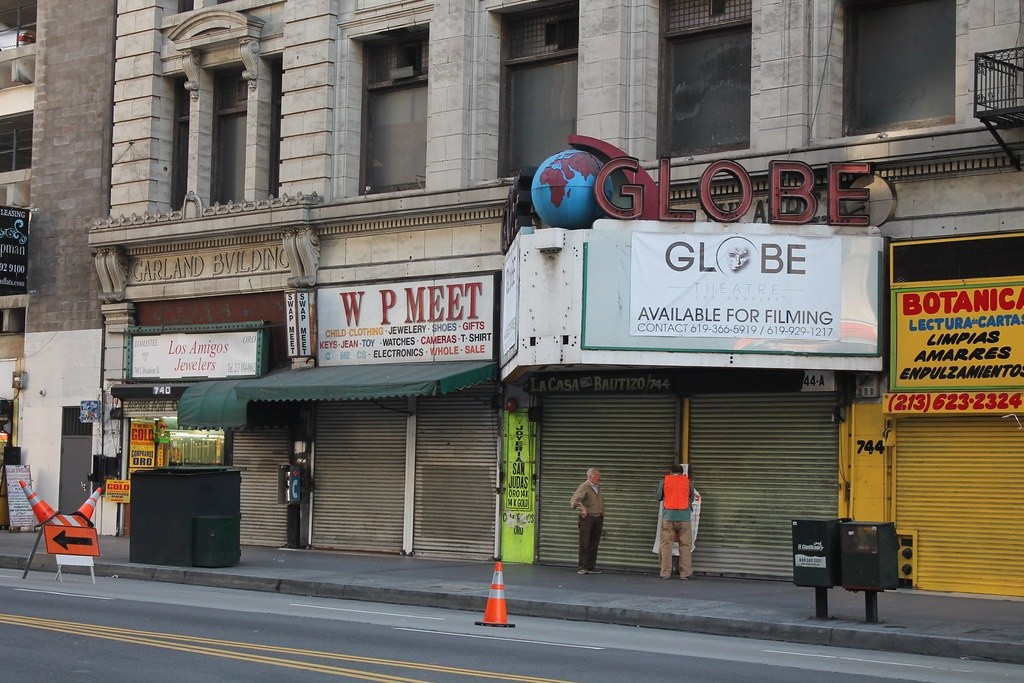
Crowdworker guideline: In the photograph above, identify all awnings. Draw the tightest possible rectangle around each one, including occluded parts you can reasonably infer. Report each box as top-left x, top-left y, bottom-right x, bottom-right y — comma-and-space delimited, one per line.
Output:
233, 361, 497, 402
176, 380, 276, 433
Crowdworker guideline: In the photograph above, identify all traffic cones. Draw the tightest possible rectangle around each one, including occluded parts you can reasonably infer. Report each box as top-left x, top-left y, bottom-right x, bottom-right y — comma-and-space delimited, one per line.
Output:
19, 480, 59, 527
69, 487, 102, 527
476, 557, 517, 629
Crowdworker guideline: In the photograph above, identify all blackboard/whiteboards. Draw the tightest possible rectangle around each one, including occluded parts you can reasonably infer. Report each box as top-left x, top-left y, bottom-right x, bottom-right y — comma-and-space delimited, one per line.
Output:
5, 464, 36, 529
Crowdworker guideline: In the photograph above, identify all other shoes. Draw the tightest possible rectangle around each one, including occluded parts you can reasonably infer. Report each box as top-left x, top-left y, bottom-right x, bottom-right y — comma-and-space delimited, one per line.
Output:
578, 570, 588, 574
588, 569, 603, 573
661, 577, 671, 579
680, 577, 689, 580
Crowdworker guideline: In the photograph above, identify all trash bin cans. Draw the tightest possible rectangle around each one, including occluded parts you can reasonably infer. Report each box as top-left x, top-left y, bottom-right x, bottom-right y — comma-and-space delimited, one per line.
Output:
128, 467, 242, 569
841, 521, 902, 591
793, 517, 851, 589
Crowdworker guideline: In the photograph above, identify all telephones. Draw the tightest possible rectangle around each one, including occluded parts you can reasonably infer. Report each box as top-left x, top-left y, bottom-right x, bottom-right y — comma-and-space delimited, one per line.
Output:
284, 472, 292, 489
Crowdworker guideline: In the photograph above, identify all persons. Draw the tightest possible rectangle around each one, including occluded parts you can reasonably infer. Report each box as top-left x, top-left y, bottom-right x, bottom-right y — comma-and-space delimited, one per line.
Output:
655, 463, 695, 581
570, 467, 605, 575
